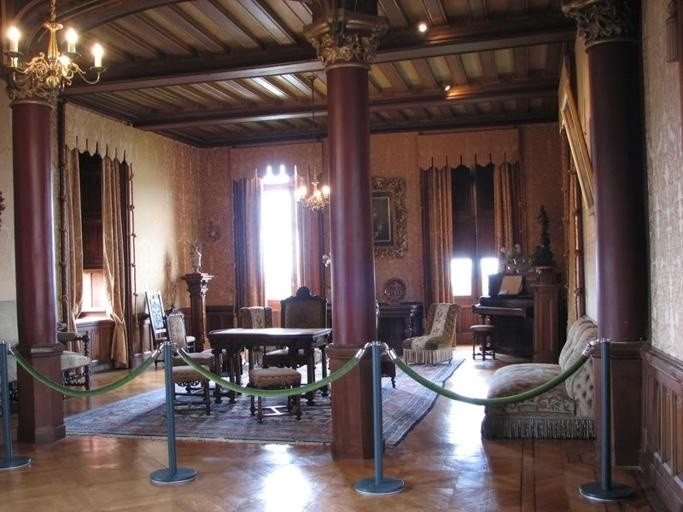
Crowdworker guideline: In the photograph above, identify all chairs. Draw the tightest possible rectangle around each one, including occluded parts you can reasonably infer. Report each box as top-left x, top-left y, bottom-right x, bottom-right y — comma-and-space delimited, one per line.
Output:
241, 306, 280, 364
261, 288, 328, 372
57, 331, 91, 398
401, 302, 459, 366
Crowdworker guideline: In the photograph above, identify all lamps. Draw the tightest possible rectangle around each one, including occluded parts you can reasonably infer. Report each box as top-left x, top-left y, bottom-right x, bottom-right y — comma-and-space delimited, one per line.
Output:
296, 76, 330, 214
0, 0, 106, 100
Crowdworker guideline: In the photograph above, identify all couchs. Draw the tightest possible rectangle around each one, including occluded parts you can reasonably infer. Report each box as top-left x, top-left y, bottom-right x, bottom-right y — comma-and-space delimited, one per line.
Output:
481, 316, 599, 439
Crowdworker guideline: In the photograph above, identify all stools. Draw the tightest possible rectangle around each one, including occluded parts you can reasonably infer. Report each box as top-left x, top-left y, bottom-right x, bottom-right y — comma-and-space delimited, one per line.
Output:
470, 325, 497, 360
173, 353, 222, 394
201, 348, 242, 388
249, 368, 302, 423
169, 364, 211, 417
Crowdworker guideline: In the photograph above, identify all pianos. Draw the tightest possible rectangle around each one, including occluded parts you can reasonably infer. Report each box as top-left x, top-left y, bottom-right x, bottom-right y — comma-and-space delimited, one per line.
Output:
472, 265, 554, 357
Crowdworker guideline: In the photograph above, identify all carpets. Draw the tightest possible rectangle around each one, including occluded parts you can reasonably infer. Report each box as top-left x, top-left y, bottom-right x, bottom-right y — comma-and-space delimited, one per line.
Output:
63, 358, 465, 449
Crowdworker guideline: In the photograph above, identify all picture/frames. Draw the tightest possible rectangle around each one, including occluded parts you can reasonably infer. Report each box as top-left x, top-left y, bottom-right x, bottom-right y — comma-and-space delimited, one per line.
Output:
146, 291, 167, 334
559, 84, 597, 216
370, 175, 408, 261
